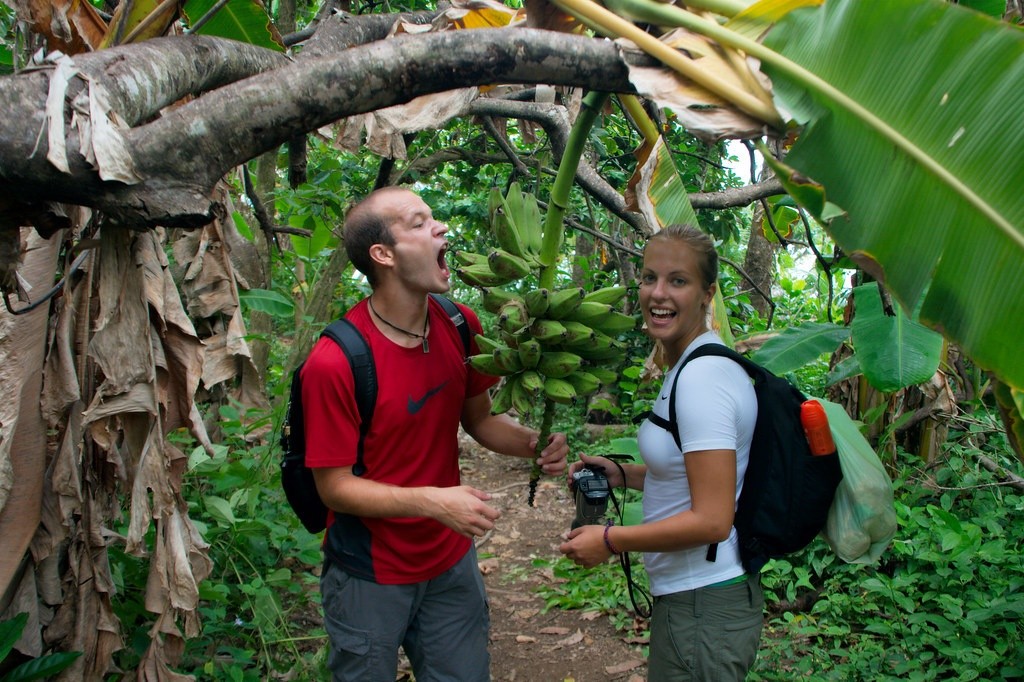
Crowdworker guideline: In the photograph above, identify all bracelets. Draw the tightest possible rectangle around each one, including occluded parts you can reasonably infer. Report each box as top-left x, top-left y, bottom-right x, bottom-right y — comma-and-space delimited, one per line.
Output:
604, 517, 622, 555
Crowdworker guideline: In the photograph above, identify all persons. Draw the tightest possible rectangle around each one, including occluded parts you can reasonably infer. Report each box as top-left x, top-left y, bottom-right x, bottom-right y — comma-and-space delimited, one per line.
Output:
300, 186, 571, 682
560, 224, 765, 682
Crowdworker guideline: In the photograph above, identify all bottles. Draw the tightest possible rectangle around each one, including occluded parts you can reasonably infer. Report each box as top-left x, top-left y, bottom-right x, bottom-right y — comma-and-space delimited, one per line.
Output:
800, 400, 835, 456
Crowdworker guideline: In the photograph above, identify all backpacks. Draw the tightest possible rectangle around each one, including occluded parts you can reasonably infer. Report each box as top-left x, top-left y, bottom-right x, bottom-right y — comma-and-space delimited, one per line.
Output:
280, 295, 471, 535
632, 343, 843, 577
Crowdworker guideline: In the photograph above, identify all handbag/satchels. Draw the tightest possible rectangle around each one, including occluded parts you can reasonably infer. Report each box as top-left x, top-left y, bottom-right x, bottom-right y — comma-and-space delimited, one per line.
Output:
800, 389, 896, 564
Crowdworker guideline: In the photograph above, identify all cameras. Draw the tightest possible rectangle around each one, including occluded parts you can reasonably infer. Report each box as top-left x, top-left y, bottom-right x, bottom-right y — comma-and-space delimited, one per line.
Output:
570, 467, 610, 533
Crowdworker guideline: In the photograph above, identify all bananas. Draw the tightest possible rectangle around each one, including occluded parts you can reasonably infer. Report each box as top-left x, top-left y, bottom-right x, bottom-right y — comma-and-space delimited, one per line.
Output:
456, 182, 638, 416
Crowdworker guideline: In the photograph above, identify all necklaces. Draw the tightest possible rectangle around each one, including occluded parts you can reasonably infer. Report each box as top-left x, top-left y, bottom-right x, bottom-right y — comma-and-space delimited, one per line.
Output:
370, 293, 429, 353
